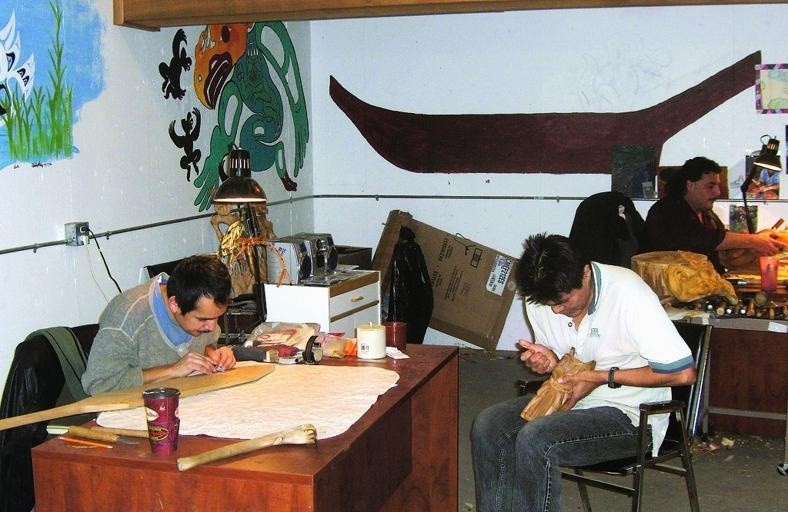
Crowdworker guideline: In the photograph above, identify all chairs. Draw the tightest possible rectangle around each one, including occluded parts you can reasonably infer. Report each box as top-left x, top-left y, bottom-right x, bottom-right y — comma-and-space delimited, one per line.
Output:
567, 187, 645, 262
515, 317, 718, 511
5, 323, 101, 512
140, 254, 265, 349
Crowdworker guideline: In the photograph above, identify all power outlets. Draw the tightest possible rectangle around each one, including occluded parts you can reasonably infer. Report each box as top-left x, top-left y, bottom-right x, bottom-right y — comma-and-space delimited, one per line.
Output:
67, 221, 94, 249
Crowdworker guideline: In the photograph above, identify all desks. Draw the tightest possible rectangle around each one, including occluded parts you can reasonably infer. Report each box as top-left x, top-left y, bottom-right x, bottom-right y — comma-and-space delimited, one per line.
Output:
674, 260, 788, 474
27, 331, 462, 512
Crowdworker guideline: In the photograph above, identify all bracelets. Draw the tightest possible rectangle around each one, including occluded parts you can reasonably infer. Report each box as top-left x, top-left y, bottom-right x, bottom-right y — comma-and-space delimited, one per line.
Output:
301, 333, 323, 365
606, 365, 622, 388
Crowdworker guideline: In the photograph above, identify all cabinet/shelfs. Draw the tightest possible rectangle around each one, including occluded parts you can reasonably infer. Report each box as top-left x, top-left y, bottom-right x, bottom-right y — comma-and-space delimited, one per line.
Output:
262, 265, 381, 345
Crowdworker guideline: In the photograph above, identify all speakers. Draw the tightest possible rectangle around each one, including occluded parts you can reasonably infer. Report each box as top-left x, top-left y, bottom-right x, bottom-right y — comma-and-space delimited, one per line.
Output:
266, 240, 314, 285
299, 232, 338, 275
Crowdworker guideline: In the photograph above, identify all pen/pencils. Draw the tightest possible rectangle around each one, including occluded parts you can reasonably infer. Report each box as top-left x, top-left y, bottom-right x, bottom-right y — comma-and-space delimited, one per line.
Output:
58, 437, 113, 449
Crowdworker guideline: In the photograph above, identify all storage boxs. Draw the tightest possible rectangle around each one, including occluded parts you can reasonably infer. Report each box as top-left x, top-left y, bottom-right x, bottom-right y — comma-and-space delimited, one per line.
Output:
369, 208, 520, 354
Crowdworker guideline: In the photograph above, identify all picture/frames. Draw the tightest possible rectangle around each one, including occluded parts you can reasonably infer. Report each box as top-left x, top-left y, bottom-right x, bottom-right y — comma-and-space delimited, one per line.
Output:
751, 61, 786, 115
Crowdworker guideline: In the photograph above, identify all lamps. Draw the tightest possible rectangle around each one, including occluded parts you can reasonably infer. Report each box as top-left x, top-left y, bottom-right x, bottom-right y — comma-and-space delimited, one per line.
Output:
739, 134, 785, 232
212, 138, 268, 328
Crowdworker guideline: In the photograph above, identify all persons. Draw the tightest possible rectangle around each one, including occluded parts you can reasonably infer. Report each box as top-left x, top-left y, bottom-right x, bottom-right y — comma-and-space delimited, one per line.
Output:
753, 167, 780, 194
645, 154, 787, 273
78, 251, 237, 397
470, 228, 699, 512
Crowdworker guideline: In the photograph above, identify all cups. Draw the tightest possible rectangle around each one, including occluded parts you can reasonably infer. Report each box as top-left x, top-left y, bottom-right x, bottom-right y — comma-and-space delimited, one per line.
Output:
357, 325, 386, 359
759, 256, 779, 290
142, 388, 180, 454
385, 322, 407, 351
